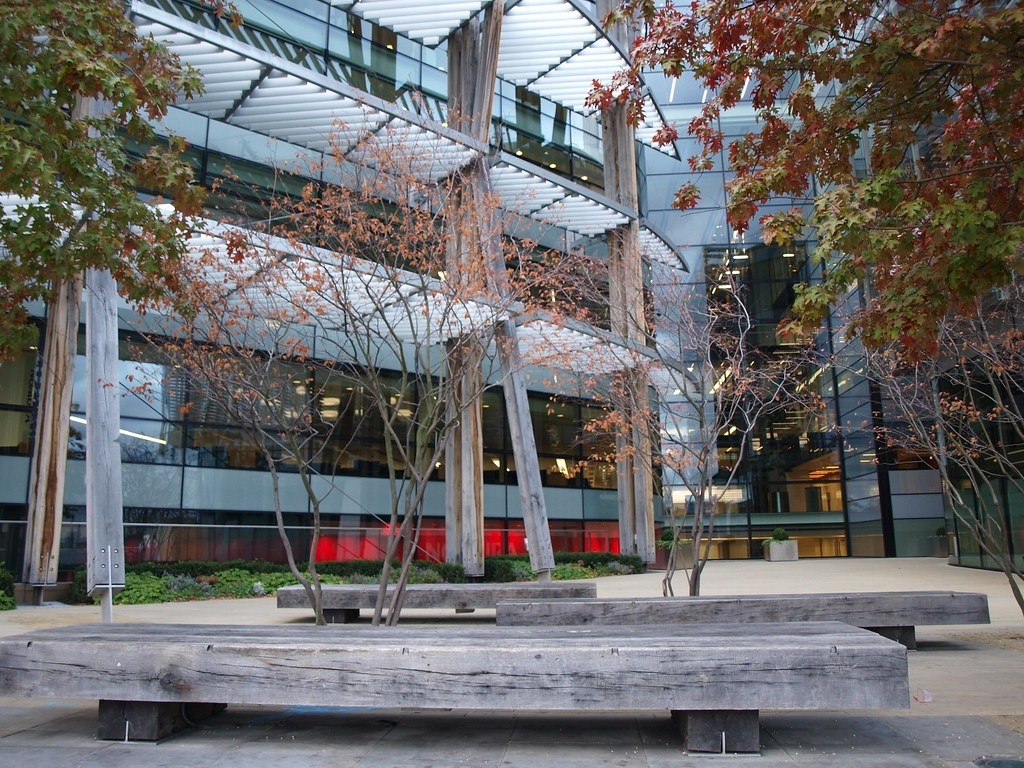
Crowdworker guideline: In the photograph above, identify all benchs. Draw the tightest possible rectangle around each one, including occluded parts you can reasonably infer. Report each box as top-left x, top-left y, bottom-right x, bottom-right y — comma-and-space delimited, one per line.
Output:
495, 592, 991, 650
277, 582, 597, 625
0, 622, 914, 754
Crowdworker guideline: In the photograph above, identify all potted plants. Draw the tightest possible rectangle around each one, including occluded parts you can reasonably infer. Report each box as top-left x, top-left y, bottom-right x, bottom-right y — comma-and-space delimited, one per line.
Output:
658, 529, 693, 569
762, 528, 799, 562
